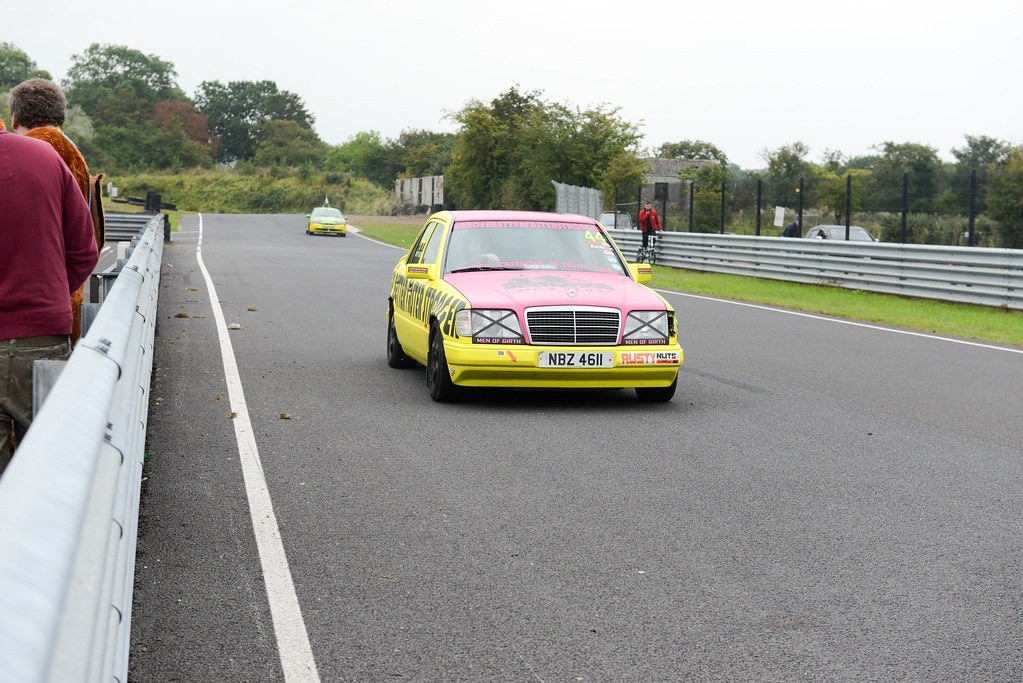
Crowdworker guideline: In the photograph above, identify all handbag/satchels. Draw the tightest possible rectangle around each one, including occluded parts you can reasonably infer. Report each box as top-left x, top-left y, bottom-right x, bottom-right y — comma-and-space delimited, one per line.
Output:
89, 173, 106, 253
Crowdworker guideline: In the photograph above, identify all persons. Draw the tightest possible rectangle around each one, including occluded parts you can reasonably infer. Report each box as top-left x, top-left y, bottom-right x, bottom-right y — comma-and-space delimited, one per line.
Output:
0, 127, 99, 480
8, 79, 95, 353
780, 217, 799, 238
638, 200, 663, 256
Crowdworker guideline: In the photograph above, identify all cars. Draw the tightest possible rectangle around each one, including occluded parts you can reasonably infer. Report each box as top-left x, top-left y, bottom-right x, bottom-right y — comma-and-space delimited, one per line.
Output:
304, 205, 348, 236
804, 225, 878, 244
386, 210, 683, 403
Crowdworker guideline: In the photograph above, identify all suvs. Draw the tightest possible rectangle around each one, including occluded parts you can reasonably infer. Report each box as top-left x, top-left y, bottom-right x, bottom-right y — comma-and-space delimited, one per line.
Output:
596, 212, 634, 230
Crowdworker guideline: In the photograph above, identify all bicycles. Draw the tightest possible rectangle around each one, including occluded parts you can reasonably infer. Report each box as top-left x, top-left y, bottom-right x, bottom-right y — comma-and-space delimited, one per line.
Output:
632, 234, 663, 264
